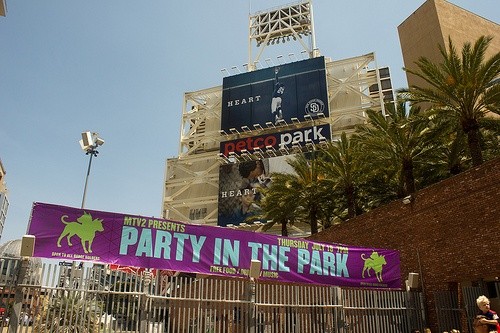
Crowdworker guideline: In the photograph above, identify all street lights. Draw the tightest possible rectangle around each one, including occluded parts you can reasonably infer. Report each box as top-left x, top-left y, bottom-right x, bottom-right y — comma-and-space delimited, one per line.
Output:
78, 131, 105, 208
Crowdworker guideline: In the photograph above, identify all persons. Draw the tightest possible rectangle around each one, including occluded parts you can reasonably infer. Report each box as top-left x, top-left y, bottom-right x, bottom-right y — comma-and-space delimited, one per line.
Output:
0, 311, 34, 327
473, 315, 493, 333
411, 327, 461, 333
271, 67, 284, 120
477, 295, 500, 333
